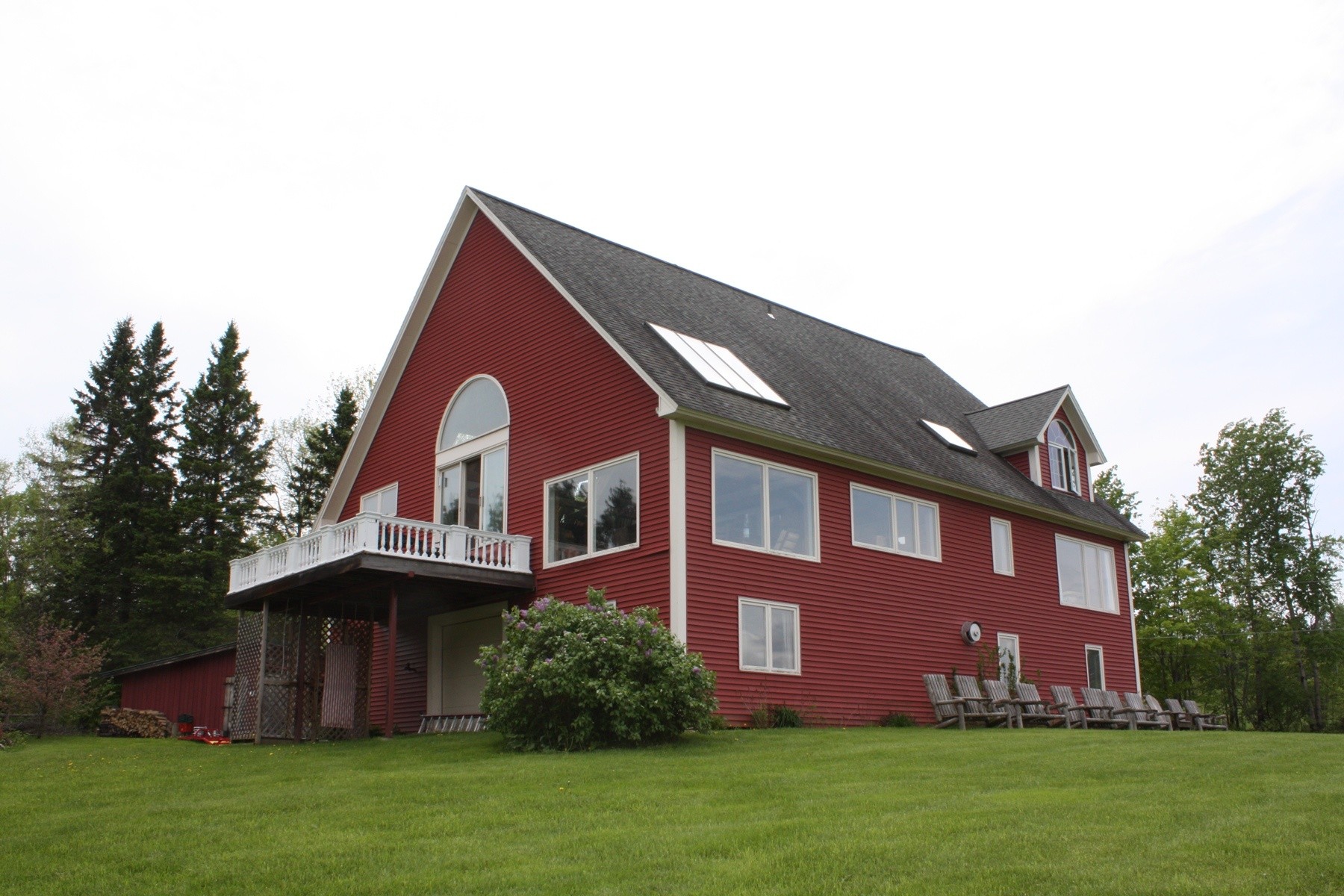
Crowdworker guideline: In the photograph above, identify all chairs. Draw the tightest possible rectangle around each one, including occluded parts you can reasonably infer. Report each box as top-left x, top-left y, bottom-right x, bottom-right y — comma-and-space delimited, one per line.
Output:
922, 671, 1230, 733
